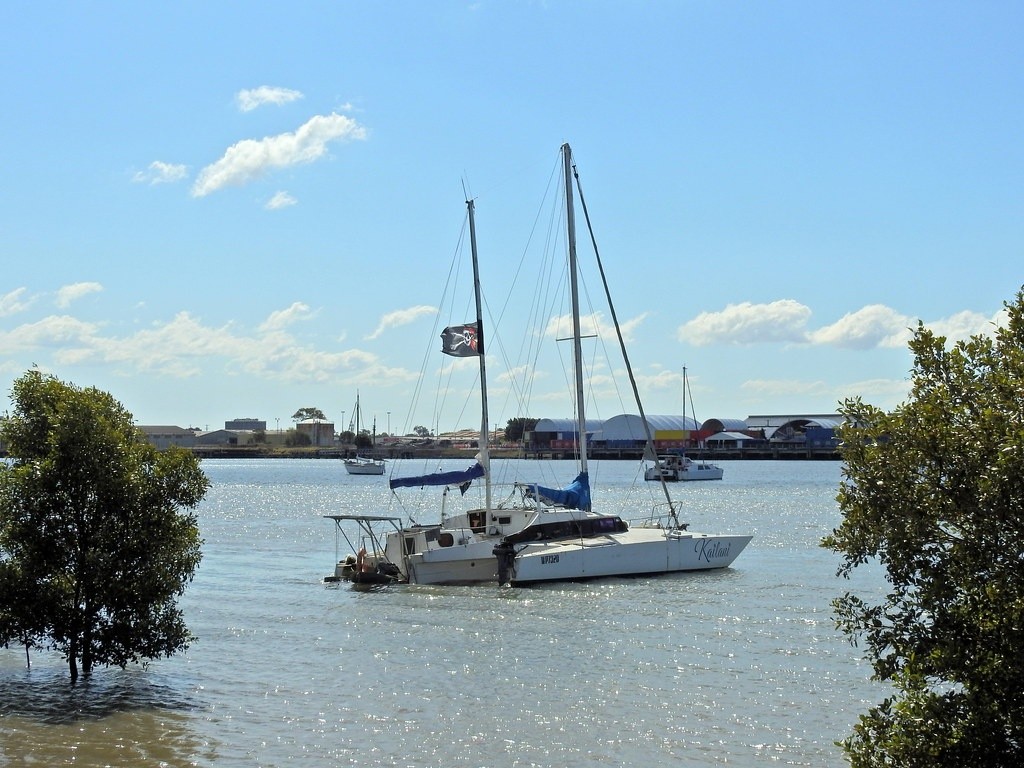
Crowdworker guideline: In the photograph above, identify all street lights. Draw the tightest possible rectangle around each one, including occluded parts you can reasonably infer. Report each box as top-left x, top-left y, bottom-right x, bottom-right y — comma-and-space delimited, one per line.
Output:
386, 412, 391, 435
275, 417, 280, 438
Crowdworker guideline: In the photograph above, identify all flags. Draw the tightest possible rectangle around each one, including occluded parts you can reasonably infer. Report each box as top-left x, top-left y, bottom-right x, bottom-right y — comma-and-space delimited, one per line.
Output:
440, 322, 480, 357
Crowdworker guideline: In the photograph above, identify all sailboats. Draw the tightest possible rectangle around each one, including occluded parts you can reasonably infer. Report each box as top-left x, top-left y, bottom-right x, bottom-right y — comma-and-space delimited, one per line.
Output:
322, 138, 763, 590
643, 362, 726, 483
340, 410, 345, 432
341, 389, 388, 476
